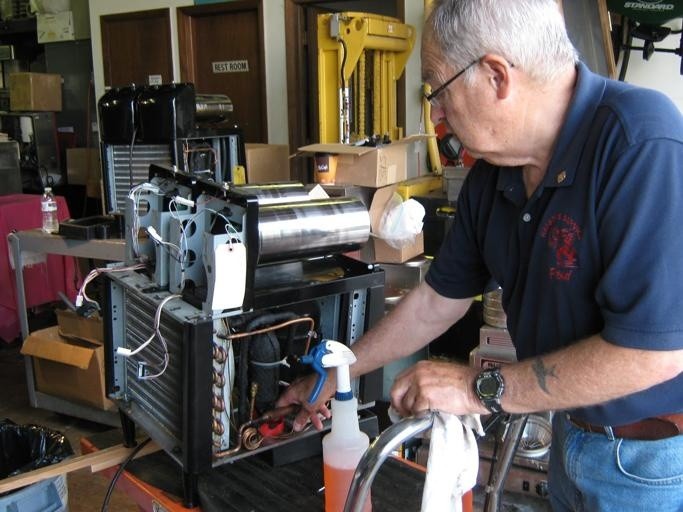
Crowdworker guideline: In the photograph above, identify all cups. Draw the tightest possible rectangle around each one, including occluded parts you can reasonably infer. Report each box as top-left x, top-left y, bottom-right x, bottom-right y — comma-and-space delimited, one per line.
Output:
314, 151, 339, 185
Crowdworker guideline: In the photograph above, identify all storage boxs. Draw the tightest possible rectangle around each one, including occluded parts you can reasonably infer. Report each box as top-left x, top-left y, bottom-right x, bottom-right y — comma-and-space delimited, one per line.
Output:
65, 148, 101, 185
36, 8, 91, 44
245, 142, 291, 184
296, 134, 438, 188
8, 71, 64, 112
365, 189, 426, 265
19, 324, 116, 413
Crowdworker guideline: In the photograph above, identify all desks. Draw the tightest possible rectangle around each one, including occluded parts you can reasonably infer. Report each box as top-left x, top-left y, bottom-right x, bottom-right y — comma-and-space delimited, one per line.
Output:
0, 193, 75, 328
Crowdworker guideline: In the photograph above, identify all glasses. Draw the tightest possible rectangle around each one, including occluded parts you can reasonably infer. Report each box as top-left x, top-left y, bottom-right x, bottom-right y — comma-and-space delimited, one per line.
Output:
423, 55, 515, 107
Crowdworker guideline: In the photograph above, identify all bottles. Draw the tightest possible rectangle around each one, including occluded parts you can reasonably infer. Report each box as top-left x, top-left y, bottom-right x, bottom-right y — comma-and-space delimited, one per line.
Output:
40, 187, 59, 232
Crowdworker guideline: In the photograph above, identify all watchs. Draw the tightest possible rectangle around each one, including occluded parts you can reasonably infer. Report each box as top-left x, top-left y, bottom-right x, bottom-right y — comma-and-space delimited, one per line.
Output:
472, 366, 510, 414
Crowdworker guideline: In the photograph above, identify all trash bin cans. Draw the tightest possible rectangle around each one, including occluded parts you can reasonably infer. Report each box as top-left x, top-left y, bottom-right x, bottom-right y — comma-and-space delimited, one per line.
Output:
0, 425, 69, 512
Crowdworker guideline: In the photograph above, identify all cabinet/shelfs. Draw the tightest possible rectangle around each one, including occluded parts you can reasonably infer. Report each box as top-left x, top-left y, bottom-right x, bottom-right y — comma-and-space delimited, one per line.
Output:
7, 219, 142, 433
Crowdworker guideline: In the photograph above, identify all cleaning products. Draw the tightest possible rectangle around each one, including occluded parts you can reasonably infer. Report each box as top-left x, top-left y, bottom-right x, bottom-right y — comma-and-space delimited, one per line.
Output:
299, 338, 373, 512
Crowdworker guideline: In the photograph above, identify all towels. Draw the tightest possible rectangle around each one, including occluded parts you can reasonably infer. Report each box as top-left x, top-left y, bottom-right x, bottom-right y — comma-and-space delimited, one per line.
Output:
387, 397, 486, 512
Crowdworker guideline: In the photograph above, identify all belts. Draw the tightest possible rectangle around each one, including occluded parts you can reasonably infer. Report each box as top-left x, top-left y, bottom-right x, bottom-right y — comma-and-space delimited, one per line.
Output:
565, 413, 683, 440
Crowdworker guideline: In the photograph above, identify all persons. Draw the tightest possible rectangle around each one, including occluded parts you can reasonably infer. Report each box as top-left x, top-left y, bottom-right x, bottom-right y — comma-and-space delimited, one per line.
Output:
275, 0, 681, 511
